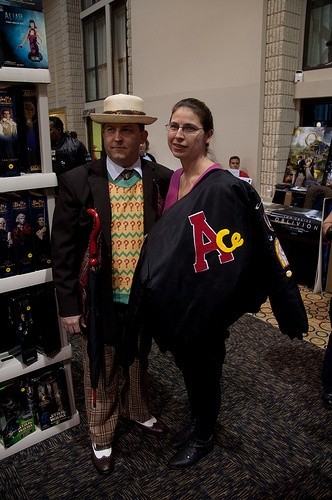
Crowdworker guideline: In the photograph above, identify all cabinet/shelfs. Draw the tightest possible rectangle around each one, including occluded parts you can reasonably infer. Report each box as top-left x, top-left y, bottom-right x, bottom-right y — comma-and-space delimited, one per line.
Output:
0, 67, 82, 461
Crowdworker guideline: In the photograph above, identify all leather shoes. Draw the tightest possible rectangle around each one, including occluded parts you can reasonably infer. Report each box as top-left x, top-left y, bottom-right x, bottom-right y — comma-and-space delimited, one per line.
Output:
90, 440, 120, 475
167, 434, 214, 469
172, 418, 197, 448
131, 409, 169, 435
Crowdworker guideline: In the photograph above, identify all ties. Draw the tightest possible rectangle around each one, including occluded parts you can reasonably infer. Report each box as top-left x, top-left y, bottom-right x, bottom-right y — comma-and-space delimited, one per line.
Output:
122, 170, 133, 180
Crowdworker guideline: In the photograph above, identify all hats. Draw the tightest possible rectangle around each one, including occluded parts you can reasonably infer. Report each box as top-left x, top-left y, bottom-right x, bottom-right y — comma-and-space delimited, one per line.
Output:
86, 88, 159, 125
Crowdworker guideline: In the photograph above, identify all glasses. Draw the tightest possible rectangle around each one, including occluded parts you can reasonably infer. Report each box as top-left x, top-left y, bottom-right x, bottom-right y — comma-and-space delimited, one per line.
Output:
164, 122, 206, 135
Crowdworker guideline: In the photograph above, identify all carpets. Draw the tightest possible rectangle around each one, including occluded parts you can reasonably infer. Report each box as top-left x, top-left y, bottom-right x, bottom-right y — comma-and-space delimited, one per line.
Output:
0, 314, 332, 500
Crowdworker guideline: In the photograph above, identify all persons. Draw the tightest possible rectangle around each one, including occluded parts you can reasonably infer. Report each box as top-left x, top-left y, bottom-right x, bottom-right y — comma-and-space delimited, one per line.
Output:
287, 156, 317, 187
51, 93, 175, 475
49, 116, 91, 176
17, 20, 43, 62
0, 213, 46, 250
163, 98, 233, 471
0, 109, 17, 136
139, 140, 156, 163
227, 156, 253, 186
